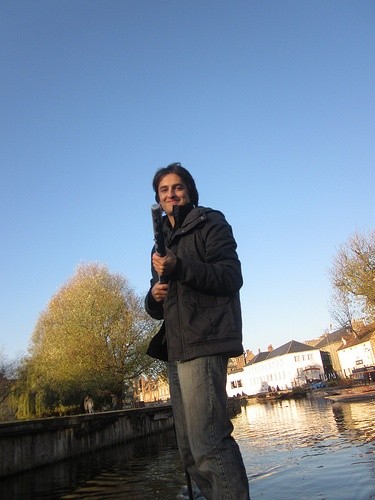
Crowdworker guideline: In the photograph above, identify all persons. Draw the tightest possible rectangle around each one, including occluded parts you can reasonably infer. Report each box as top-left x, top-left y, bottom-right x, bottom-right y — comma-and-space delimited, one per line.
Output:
83, 395, 94, 414
233, 383, 290, 398
143, 163, 252, 500
109, 392, 118, 410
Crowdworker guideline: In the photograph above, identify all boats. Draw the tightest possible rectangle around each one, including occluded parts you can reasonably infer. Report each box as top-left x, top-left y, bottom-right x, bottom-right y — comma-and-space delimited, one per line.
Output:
253, 366, 375, 403
